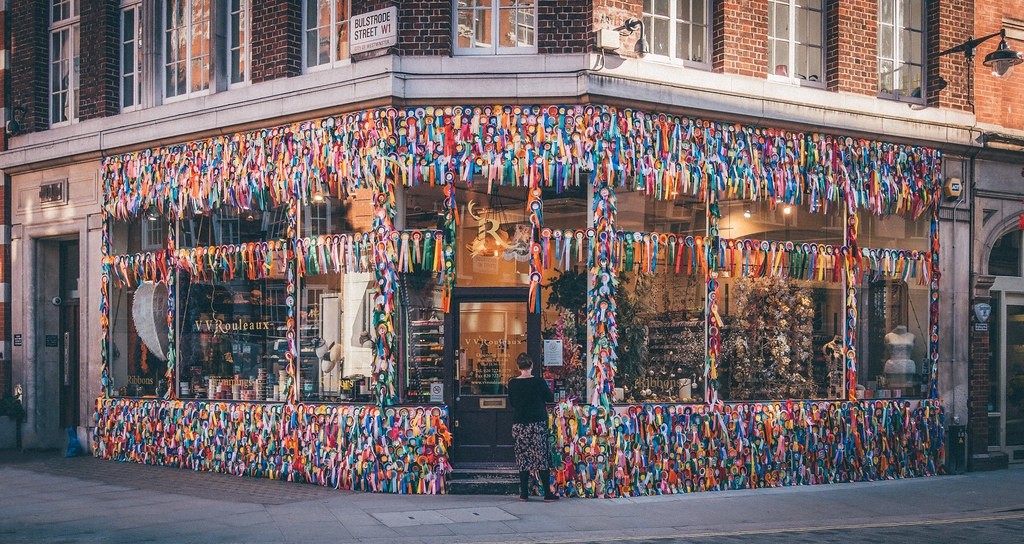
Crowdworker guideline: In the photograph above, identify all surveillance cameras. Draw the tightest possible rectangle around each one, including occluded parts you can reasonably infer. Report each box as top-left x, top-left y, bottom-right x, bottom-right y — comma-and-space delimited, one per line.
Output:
634, 38, 650, 58
7, 121, 19, 137
52, 297, 61, 305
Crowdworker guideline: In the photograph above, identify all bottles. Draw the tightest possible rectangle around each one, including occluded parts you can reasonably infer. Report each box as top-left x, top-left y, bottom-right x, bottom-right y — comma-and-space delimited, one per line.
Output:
359, 381, 366, 395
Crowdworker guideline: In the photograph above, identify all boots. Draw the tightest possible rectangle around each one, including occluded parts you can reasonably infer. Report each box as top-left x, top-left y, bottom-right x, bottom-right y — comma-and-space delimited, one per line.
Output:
519, 471, 529, 502
539, 470, 560, 501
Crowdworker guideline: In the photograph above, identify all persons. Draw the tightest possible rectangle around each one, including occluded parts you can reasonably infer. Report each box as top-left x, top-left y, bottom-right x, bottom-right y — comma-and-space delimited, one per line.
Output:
508, 353, 560, 502
884, 325, 917, 375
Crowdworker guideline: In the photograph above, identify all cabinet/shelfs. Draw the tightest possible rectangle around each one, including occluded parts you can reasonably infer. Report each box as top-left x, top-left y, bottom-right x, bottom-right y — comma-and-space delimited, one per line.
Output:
125, 280, 922, 403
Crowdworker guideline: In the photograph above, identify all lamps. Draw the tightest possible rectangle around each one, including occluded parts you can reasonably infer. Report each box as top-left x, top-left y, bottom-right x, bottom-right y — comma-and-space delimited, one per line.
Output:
625, 18, 649, 57
147, 206, 158, 221
939, 28, 1024, 77
314, 192, 338, 203
744, 209, 752, 218
776, 195, 783, 204
